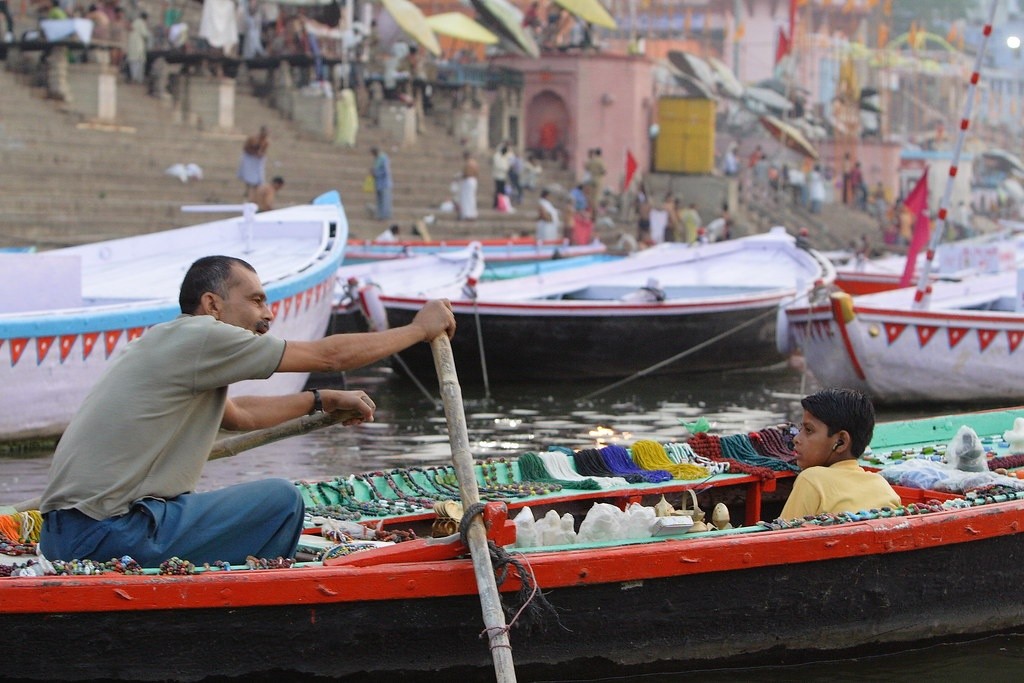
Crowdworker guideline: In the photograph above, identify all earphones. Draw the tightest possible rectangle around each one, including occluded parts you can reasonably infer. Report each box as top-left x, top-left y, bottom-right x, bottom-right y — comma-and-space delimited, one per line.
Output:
831, 439, 844, 452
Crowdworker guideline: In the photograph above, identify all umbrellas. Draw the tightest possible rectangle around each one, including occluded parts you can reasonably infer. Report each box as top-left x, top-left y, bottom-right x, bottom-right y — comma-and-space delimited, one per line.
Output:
761, 115, 819, 162
428, 10, 498, 65
475, 0, 538, 57
668, 50, 718, 100
673, 73, 710, 98
553, 0, 617, 28
748, 87, 794, 110
381, 0, 440, 62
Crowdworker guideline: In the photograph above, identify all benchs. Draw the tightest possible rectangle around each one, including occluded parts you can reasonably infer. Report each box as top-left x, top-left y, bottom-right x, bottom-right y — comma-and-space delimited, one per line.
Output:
300, 462, 791, 536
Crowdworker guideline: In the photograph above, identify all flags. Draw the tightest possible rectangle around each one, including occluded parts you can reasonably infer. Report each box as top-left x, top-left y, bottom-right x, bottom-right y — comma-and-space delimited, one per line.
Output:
906, 180, 931, 246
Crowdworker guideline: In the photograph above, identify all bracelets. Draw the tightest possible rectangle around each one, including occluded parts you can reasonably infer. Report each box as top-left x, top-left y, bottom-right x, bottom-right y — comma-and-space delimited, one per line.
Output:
308, 388, 323, 411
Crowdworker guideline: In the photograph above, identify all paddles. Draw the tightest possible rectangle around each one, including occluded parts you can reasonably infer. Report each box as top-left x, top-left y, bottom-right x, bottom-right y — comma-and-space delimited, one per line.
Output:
0, 397, 371, 514
431, 334, 516, 683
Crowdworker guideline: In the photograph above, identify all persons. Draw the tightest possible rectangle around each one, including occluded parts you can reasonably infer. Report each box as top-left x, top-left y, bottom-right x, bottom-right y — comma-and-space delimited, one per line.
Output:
38, 255, 456, 565
780, 388, 902, 519
1, 1, 969, 254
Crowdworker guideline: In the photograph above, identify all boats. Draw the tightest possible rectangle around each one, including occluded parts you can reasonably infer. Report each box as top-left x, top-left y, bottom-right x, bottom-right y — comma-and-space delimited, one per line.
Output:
1, 407, 1024, 663
346, 243, 607, 279
0, 191, 348, 444
327, 245, 483, 334
342, 229, 839, 380
788, 262, 1024, 406
831, 262, 967, 294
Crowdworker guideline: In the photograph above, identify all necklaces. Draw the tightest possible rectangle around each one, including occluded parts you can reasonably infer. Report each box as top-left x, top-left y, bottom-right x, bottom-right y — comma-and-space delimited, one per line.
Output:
294, 423, 799, 521
0, 510, 418, 576
805, 491, 1018, 524
